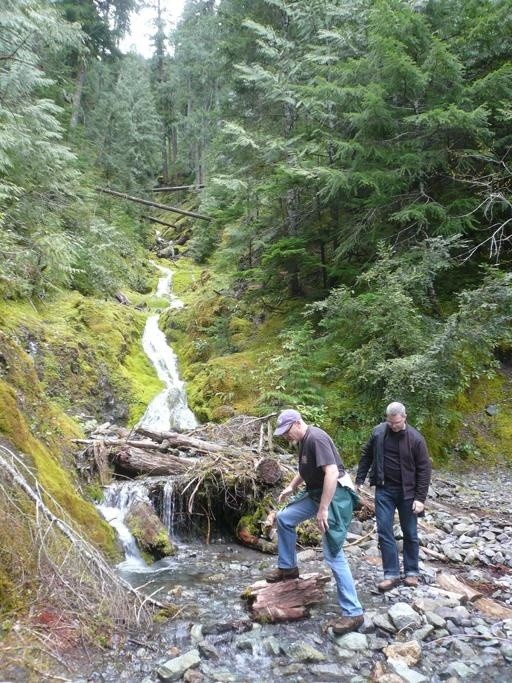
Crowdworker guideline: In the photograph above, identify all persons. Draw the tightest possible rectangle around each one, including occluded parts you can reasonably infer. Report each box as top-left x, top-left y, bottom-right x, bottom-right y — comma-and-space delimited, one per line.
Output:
354, 402, 431, 592
264, 408, 365, 637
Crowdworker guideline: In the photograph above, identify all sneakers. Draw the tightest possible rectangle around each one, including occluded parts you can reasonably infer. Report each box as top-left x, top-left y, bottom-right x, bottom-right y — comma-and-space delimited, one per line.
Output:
332, 614, 364, 635
266, 566, 300, 583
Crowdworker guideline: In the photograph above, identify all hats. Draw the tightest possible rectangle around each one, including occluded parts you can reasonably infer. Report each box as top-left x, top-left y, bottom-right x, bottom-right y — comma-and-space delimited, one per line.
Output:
272, 409, 301, 438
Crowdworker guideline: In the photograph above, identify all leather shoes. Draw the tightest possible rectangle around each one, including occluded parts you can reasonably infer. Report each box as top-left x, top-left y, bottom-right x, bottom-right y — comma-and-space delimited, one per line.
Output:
378, 577, 401, 592
403, 574, 419, 586
